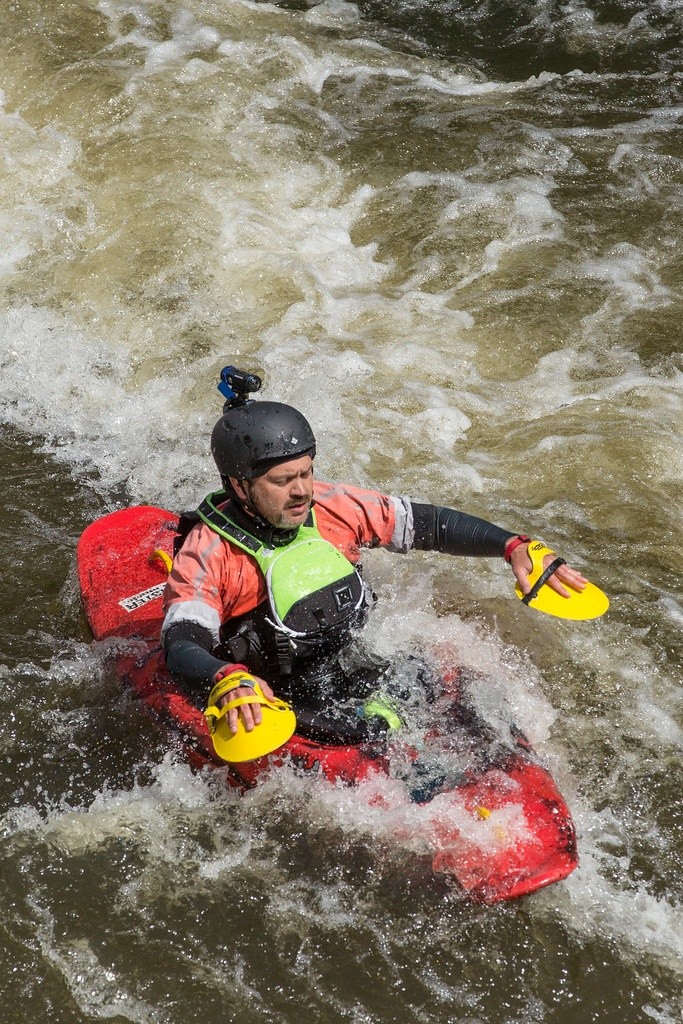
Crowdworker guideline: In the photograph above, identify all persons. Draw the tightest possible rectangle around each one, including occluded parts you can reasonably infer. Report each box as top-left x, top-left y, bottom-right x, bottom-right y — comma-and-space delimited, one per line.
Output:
159, 400, 589, 737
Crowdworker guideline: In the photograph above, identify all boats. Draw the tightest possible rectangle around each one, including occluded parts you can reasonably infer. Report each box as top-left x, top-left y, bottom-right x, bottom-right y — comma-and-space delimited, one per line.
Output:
77, 505, 580, 904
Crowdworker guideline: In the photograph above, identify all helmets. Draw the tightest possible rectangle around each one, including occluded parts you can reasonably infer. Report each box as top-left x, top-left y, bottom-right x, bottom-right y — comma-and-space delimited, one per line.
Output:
209, 400, 317, 479
